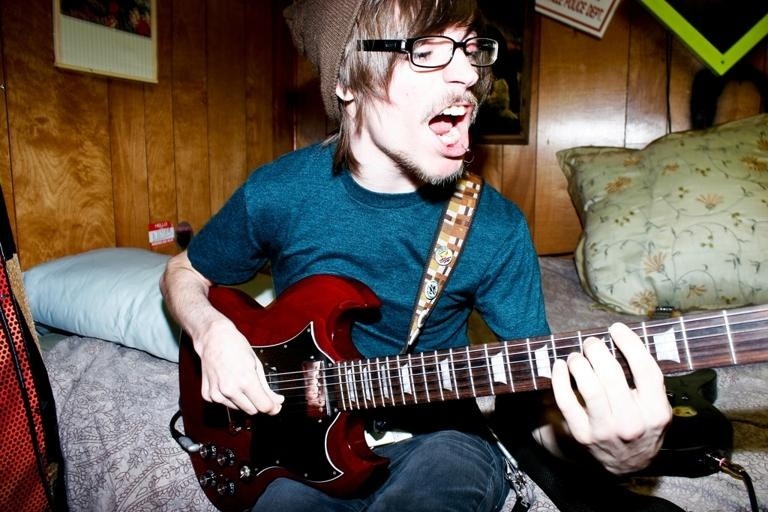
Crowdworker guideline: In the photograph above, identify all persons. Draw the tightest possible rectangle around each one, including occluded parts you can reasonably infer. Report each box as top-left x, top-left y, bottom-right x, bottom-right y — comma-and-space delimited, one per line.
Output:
159, 1, 674, 511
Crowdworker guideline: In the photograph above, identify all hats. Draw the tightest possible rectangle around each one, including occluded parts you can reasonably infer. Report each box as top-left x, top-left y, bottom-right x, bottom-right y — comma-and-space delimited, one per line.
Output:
282, 0, 363, 123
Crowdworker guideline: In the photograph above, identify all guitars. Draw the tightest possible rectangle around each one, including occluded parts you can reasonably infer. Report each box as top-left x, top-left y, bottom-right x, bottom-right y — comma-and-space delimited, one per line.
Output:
179, 273, 768, 511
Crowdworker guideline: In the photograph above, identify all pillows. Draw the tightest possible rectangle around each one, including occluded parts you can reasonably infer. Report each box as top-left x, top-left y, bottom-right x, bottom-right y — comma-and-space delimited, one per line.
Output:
557, 112, 768, 318
18, 246, 183, 366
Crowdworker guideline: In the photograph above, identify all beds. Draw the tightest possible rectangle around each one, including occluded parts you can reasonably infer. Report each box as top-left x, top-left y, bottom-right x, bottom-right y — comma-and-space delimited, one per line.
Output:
30, 253, 768, 511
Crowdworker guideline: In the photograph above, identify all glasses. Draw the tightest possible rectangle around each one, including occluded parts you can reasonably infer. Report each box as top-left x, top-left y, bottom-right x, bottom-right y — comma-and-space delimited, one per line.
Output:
357, 36, 499, 68
